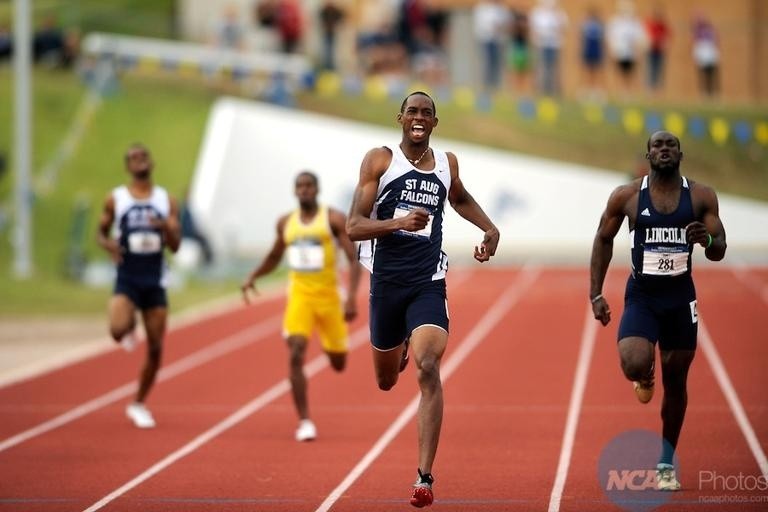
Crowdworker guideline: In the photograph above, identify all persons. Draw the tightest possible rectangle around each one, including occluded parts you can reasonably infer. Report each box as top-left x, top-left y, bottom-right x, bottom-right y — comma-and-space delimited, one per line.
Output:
93, 143, 182, 429
240, 1, 564, 101
693, 18, 721, 98
240, 170, 362, 440
583, 0, 669, 92
587, 131, 727, 496
0, 15, 90, 71
340, 90, 500, 509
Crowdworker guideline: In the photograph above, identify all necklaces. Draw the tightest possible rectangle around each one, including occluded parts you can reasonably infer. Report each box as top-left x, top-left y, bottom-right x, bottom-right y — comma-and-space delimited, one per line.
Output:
398, 145, 432, 167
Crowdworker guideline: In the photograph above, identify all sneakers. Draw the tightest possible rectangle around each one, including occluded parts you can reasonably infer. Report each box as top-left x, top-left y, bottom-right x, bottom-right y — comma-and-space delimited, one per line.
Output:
125, 404, 155, 429
296, 421, 315, 441
409, 473, 433, 508
653, 464, 680, 491
632, 361, 654, 403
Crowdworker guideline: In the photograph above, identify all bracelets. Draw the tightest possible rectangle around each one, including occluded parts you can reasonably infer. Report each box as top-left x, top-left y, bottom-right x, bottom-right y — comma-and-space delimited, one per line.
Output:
700, 232, 712, 248
590, 294, 605, 305
